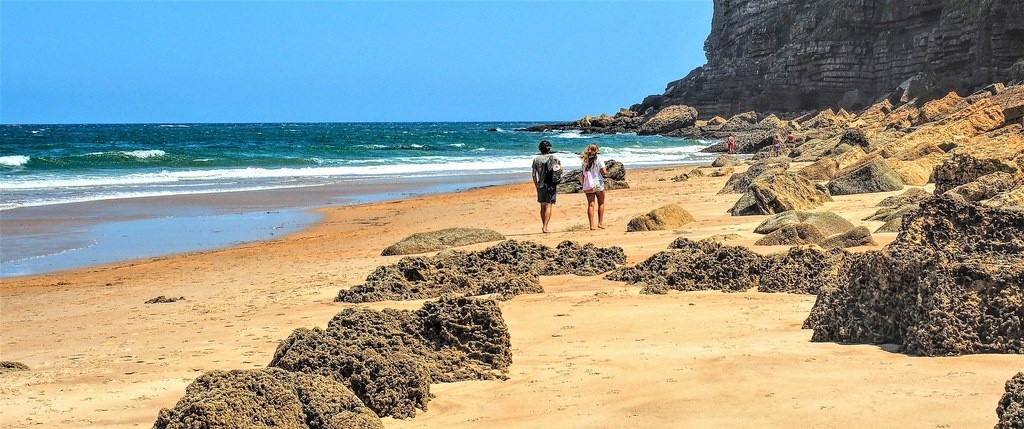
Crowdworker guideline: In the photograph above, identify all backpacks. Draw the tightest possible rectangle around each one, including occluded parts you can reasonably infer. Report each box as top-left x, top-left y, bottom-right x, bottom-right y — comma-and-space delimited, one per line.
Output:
583, 171, 595, 191
537, 155, 563, 185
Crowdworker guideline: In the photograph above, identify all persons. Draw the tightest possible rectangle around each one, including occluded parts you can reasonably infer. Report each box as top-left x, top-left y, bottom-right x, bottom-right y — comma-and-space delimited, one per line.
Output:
580, 143, 607, 231
786, 131, 796, 144
728, 132, 735, 155
774, 134, 781, 157
531, 140, 563, 233
